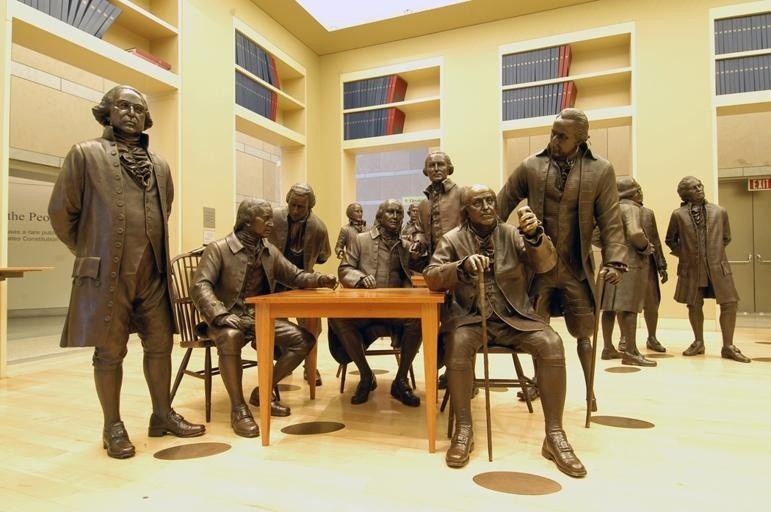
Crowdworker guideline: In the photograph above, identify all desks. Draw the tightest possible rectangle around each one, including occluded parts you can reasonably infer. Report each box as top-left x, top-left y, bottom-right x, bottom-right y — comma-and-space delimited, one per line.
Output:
411, 275, 429, 288
244, 287, 447, 453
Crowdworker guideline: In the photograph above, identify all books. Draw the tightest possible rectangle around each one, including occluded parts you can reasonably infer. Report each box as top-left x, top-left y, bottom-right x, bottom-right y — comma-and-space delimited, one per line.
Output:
502, 44, 578, 120
17, 0, 172, 70
234, 32, 281, 122
344, 75, 408, 140
714, 14, 771, 95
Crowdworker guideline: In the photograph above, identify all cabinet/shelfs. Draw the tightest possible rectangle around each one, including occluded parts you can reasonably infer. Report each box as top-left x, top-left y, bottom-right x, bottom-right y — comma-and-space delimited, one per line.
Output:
710, 0, 770, 109
341, 57, 441, 151
233, 18, 307, 148
6, 0, 181, 90
498, 20, 634, 132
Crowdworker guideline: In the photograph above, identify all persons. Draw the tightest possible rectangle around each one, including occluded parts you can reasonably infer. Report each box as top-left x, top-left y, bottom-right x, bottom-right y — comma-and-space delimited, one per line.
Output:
47, 85, 206, 458
330, 152, 473, 406
496, 108, 629, 402
425, 186, 587, 478
189, 185, 340, 436
666, 176, 749, 363
591, 176, 668, 367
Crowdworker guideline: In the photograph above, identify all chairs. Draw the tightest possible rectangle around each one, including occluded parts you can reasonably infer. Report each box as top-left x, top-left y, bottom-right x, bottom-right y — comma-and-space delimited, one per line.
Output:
169, 253, 281, 423
440, 345, 541, 413
336, 334, 416, 393
